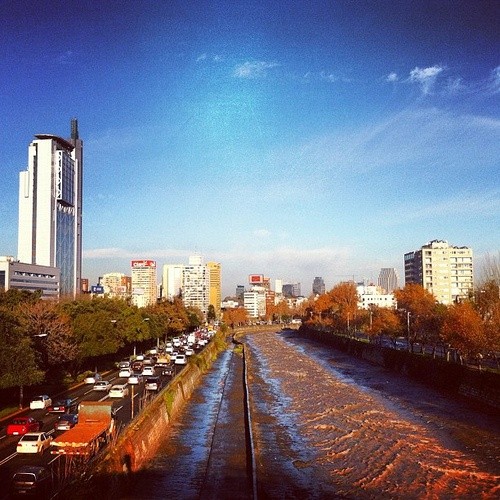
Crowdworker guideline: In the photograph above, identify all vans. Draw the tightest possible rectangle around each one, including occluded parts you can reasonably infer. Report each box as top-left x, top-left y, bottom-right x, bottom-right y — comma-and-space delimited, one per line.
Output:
7, 467, 50, 499
144, 377, 163, 393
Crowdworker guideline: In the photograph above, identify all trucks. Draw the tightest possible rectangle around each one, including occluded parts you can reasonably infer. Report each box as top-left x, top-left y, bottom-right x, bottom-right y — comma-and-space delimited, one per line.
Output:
50, 400, 120, 464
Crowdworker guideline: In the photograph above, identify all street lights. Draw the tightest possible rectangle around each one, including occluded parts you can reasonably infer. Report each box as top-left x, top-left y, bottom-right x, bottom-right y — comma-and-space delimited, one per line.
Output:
19, 333, 48, 410
134, 318, 150, 356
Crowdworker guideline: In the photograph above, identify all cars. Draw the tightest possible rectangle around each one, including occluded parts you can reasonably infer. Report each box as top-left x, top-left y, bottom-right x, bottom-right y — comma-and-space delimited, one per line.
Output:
30, 394, 52, 410
396, 337, 407, 349
119, 328, 217, 378
47, 398, 78, 414
109, 384, 129, 398
54, 414, 78, 431
93, 380, 113, 392
15, 431, 54, 456
84, 372, 102, 385
6, 416, 41, 437
